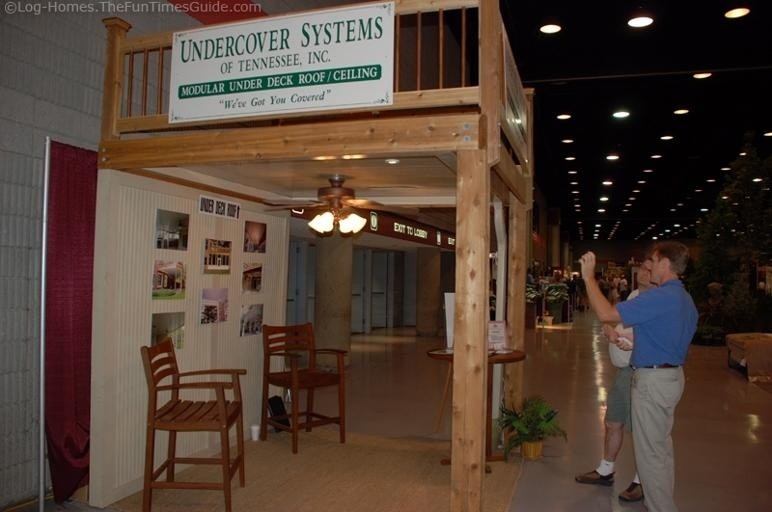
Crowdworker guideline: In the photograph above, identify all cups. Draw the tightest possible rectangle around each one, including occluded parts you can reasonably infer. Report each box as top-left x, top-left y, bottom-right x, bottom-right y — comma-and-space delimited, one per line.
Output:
250, 425, 259, 442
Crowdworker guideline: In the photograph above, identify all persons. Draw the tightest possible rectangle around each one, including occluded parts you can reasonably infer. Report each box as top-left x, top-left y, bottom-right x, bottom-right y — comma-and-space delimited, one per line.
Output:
575, 257, 658, 502
564, 273, 628, 312
527, 265, 552, 285
579, 241, 700, 512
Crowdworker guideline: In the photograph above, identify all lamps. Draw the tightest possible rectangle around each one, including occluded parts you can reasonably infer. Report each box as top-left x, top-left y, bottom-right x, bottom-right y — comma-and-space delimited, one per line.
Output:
305, 198, 367, 238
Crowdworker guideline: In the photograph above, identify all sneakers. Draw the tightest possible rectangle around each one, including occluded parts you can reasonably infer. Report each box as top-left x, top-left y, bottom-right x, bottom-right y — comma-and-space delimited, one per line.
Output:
575, 470, 615, 486
618, 482, 644, 502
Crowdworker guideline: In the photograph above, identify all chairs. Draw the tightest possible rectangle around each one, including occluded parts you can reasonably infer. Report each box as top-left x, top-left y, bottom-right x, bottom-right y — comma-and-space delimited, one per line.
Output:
258, 322, 348, 455
140, 337, 248, 512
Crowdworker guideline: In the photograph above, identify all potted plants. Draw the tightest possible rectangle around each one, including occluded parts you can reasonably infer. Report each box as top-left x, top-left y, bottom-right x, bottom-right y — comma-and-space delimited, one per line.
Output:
497, 394, 568, 466
525, 282, 570, 330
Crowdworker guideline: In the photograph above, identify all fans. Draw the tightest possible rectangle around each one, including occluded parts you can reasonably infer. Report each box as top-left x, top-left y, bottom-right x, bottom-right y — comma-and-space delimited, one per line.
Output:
261, 174, 421, 218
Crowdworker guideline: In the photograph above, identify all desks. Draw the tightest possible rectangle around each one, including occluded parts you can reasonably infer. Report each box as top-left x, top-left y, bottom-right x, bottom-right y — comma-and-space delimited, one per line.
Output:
425, 347, 527, 460
725, 332, 771, 380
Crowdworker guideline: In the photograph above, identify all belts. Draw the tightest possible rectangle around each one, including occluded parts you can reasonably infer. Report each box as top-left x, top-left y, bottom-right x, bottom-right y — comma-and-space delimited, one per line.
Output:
629, 363, 679, 371
